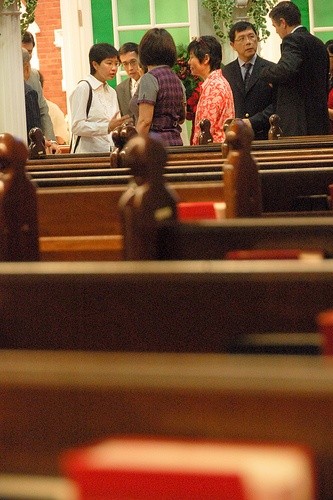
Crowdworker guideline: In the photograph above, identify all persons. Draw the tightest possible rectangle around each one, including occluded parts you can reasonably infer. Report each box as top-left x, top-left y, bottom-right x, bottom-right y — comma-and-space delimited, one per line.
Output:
135, 62, 148, 88
21, 30, 68, 154
255, 1, 330, 137
187, 35, 235, 145
116, 41, 140, 128
68, 42, 132, 153
325, 39, 333, 135
184, 81, 204, 146
129, 27, 186, 147
221, 22, 278, 141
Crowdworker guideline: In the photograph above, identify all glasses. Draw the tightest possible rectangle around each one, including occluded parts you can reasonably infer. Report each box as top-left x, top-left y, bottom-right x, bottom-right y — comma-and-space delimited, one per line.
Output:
102, 59, 120, 68
121, 59, 140, 68
235, 33, 258, 44
192, 36, 207, 49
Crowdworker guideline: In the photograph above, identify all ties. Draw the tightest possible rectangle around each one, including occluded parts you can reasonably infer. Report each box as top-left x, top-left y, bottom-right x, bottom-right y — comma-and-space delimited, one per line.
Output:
243, 63, 253, 94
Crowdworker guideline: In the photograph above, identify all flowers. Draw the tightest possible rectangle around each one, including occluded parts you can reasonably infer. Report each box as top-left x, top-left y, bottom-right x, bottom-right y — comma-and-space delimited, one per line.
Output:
173, 47, 203, 119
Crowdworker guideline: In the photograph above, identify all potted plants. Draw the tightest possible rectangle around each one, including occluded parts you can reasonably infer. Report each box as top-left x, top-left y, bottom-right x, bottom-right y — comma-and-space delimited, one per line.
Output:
201, 0, 279, 44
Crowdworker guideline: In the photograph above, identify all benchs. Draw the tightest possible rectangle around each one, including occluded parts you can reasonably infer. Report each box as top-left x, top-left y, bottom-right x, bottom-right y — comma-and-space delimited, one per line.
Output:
0, 114, 333, 500
